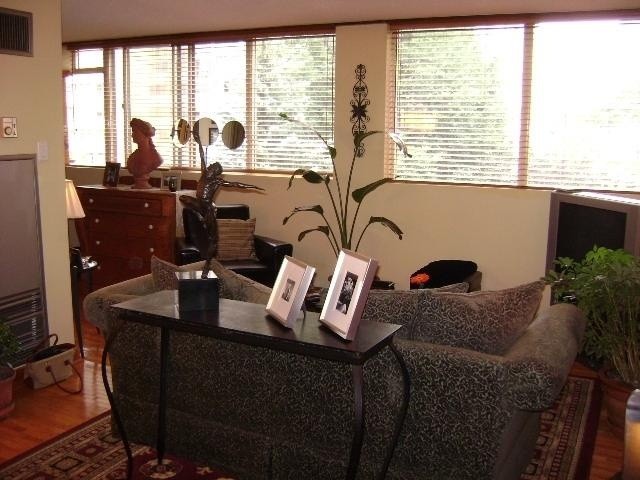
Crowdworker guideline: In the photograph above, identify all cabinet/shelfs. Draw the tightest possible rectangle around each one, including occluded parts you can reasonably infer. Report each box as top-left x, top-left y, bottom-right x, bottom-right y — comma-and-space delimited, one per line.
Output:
73, 184, 198, 298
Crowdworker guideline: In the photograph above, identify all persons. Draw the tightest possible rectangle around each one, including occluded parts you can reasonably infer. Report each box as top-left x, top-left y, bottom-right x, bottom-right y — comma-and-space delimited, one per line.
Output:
124, 116, 164, 177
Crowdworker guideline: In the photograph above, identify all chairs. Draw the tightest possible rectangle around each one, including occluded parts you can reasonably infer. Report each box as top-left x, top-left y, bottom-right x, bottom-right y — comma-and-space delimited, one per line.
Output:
177, 204, 293, 290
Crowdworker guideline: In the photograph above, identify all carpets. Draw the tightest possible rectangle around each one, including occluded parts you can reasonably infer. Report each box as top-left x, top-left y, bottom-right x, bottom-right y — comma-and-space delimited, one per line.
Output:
0, 373, 605, 479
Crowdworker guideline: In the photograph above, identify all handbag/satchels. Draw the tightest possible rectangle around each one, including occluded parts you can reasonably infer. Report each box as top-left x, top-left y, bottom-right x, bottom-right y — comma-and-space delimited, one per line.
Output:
25, 334, 83, 393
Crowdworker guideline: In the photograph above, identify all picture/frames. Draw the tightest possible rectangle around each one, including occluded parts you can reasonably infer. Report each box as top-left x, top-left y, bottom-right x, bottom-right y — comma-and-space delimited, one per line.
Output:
265, 248, 380, 344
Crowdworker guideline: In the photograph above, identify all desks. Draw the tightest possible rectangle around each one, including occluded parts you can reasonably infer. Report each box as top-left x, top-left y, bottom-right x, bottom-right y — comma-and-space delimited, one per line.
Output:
100, 286, 410, 478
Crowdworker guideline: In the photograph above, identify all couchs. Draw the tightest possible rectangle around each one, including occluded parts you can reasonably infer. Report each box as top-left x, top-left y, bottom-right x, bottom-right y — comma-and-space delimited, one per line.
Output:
82, 255, 586, 479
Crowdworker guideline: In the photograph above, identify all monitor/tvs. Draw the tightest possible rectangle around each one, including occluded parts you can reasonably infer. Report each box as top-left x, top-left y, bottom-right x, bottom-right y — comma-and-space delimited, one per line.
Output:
542, 187, 640, 285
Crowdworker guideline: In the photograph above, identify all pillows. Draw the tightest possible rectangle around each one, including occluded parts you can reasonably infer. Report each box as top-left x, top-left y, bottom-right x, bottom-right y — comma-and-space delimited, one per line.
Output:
215, 216, 260, 261
